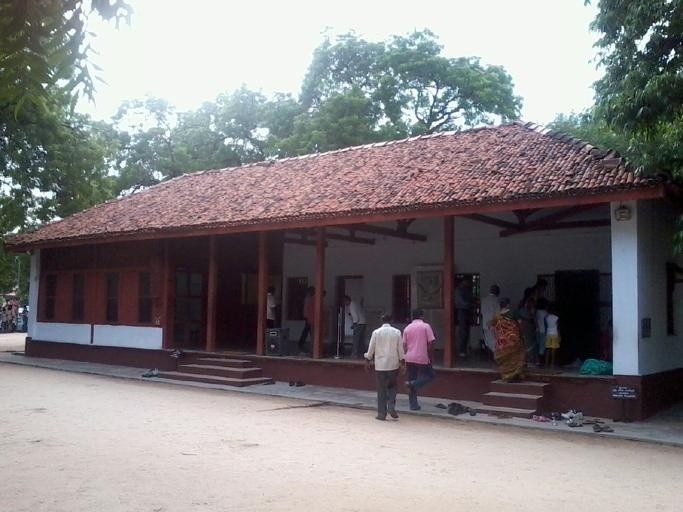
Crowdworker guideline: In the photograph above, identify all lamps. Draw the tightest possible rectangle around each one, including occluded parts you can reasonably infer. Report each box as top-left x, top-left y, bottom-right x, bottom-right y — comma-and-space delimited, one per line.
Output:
615, 201, 631, 223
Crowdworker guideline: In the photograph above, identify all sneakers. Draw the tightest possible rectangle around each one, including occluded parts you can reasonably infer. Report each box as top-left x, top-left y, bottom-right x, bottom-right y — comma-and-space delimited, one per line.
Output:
376, 416, 385, 420
404, 380, 411, 394
390, 410, 399, 419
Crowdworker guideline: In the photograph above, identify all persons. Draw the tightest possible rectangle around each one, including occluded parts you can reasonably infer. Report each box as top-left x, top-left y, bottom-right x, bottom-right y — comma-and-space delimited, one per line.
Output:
454, 275, 590, 383
401, 310, 436, 410
267, 287, 281, 328
344, 296, 367, 359
312, 289, 326, 351
0, 305, 28, 333
299, 287, 315, 349
364, 315, 405, 420
